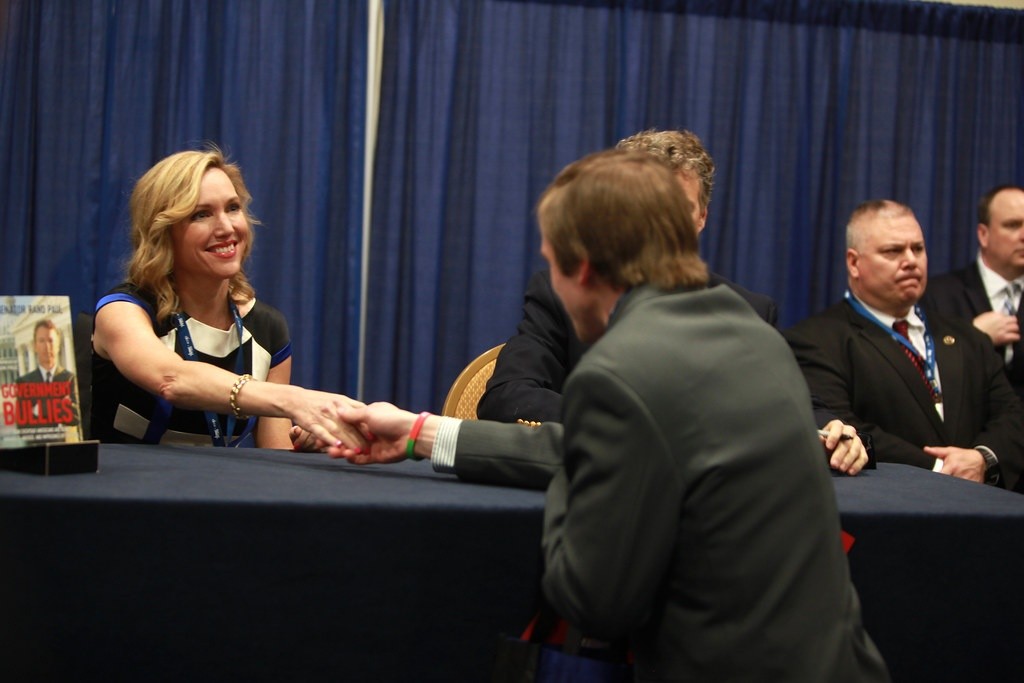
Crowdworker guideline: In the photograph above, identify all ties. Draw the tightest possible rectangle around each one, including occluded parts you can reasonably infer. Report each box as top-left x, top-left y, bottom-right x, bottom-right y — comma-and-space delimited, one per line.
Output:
999, 283, 1017, 315
44, 372, 51, 384
891, 320, 935, 406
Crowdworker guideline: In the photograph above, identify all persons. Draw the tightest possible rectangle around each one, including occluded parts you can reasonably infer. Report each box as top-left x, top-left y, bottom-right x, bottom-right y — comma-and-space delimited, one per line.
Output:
81, 150, 374, 454
785, 188, 1024, 496
329, 128, 892, 683
16, 319, 79, 429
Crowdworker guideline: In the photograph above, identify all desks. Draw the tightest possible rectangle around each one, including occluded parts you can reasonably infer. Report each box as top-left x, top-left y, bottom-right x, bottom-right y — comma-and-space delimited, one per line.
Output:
0, 443, 1024, 683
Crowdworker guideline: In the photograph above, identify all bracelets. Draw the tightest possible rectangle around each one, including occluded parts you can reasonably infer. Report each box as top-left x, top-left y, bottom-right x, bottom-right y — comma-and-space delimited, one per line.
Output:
229, 374, 252, 421
406, 411, 431, 461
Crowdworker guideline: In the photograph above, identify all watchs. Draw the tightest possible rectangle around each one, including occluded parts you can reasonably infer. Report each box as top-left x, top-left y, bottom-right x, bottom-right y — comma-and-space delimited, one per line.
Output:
976, 448, 999, 483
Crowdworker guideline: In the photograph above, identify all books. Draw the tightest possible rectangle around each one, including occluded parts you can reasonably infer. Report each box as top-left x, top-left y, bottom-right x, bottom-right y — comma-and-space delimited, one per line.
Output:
0, 296, 83, 448
